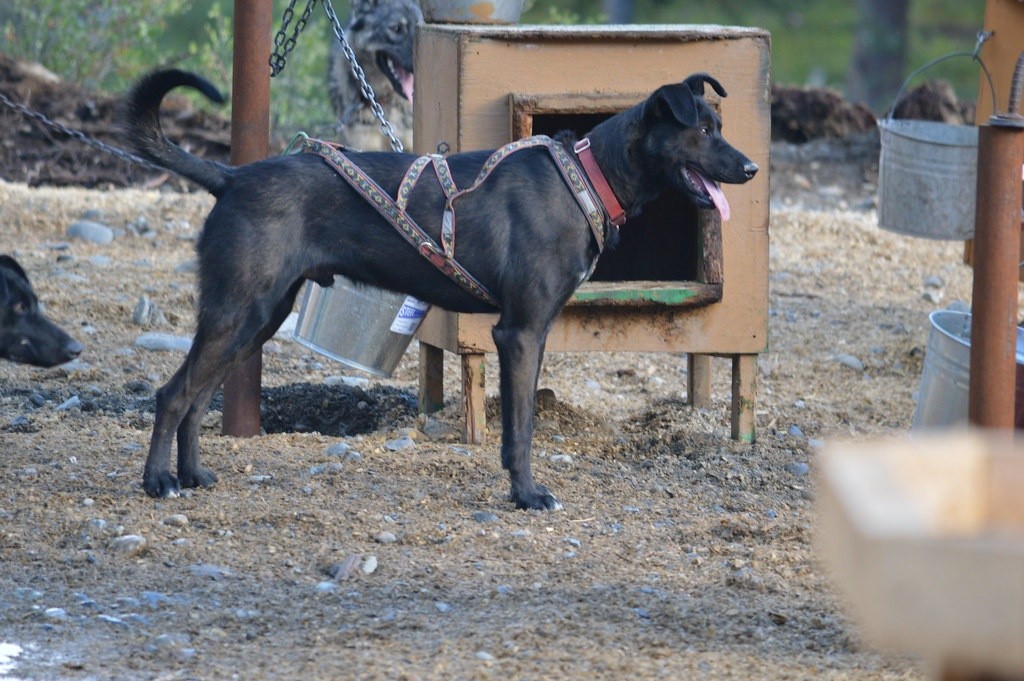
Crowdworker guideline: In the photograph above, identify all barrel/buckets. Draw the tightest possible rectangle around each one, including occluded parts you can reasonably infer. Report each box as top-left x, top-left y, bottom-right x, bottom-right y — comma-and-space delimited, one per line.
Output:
420, 0, 524, 25
912, 309, 1024, 430
294, 274, 431, 377
881, 51, 998, 240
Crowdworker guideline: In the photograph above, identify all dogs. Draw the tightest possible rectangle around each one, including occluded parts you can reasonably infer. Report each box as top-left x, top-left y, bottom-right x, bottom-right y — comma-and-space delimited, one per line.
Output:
321, 0, 426, 154
122, 70, 759, 512
0, 253, 84, 369
